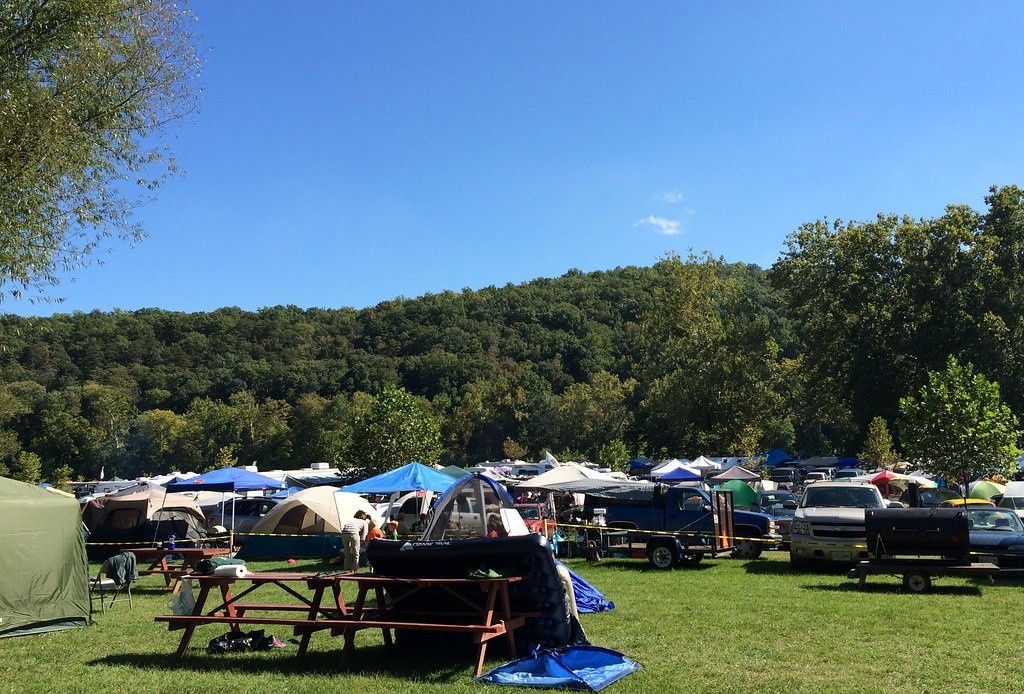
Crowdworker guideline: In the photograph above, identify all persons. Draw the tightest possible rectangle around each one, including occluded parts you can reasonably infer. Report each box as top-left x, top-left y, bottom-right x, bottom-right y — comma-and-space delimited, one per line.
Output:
341, 509, 507, 574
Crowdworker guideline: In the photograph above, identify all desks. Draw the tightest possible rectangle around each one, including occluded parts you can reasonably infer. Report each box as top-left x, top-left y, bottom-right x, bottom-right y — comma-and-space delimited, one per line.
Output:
120, 548, 230, 593
169, 572, 523, 675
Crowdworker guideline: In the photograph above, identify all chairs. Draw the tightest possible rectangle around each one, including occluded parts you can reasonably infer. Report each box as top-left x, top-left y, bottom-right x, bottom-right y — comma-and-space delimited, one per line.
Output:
89, 551, 138, 615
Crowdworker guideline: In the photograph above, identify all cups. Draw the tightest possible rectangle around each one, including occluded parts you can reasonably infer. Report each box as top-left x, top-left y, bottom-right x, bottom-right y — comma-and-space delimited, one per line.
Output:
583, 519, 587, 526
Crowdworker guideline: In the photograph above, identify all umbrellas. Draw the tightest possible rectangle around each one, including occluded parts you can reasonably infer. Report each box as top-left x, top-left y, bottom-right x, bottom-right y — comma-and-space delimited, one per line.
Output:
150, 465, 290, 530
333, 462, 472, 537
438, 459, 626, 526
624, 448, 1024, 511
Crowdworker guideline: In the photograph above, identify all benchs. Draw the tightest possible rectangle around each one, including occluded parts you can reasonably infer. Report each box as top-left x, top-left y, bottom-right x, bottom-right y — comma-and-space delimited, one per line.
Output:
156, 565, 192, 568
233, 601, 542, 619
154, 613, 503, 639
139, 570, 190, 577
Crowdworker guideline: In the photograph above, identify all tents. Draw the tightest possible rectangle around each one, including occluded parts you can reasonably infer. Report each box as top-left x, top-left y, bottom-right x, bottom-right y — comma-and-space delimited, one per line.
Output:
233, 485, 387, 559
79, 470, 197, 560
419, 470, 531, 542
471, 643, 644, 692
0, 476, 93, 639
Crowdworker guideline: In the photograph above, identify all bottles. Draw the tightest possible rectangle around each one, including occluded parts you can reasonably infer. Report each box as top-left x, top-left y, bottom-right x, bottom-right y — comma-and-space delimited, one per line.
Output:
168, 534, 175, 550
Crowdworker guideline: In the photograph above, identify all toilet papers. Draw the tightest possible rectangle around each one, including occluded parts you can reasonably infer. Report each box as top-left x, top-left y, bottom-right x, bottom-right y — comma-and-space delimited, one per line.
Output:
214, 564, 255, 577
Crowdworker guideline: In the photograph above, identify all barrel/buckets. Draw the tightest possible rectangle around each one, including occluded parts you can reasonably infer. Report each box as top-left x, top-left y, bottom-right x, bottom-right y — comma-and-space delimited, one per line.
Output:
865, 508, 970, 556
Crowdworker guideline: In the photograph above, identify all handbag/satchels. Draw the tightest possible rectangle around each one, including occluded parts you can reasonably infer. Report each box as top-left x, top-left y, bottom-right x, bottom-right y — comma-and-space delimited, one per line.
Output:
167, 574, 195, 615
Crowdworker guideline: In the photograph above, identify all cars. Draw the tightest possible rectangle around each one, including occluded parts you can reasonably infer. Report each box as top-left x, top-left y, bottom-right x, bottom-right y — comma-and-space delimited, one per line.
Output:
206, 457, 1024, 571
